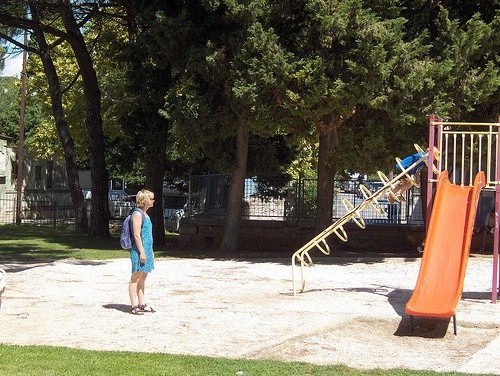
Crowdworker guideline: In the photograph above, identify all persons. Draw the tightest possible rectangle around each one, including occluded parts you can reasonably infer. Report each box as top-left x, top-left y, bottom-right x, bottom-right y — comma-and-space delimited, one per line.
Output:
385, 146, 440, 202
480, 208, 495, 251
130, 189, 156, 315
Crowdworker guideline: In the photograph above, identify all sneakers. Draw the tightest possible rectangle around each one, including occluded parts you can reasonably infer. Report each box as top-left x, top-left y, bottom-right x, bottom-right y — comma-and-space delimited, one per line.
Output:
479, 247, 488, 252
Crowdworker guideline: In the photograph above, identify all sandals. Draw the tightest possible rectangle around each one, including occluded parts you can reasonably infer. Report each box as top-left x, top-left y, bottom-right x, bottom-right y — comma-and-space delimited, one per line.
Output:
130, 306, 144, 315
138, 304, 156, 312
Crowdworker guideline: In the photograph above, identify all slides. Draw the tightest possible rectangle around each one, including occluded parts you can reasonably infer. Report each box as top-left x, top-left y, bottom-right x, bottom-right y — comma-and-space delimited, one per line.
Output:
405, 170, 486, 318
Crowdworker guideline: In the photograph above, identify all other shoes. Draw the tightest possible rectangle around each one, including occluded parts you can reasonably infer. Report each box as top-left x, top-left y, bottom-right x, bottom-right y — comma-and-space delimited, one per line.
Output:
384, 191, 394, 204
395, 193, 405, 201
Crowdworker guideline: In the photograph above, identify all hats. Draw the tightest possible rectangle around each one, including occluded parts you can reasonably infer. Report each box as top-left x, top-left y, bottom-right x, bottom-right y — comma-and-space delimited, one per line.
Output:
426, 146, 440, 161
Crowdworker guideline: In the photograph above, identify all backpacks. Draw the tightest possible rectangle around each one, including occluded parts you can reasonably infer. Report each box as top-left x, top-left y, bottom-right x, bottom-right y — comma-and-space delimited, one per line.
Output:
120, 208, 144, 250
